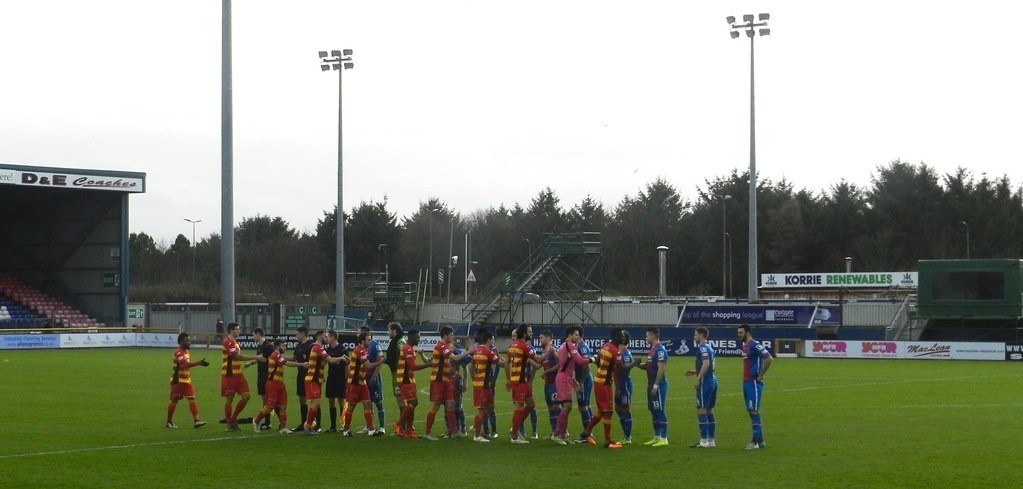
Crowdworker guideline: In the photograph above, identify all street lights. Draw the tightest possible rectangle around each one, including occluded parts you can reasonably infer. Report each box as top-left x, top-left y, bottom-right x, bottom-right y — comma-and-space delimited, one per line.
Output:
716, 194, 732, 298
962, 222, 970, 259
319, 49, 352, 330
727, 13, 771, 303
184, 219, 203, 303
379, 244, 388, 314
525, 238, 532, 272
428, 208, 439, 301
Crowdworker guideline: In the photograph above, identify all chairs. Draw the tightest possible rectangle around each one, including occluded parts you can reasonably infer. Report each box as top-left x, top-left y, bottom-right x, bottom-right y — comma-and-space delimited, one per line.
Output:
0, 275, 105, 328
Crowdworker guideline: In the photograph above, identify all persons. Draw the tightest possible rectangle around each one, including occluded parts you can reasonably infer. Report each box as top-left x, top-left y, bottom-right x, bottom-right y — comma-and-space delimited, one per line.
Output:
242, 327, 282, 430
738, 324, 773, 450
283, 326, 322, 433
253, 339, 309, 435
166, 333, 209, 429
42, 321, 52, 334
684, 327, 717, 448
380, 322, 541, 443
340, 332, 386, 436
636, 328, 668, 447
220, 322, 265, 432
304, 329, 348, 433
539, 325, 642, 448
356, 326, 385, 435
324, 330, 348, 434
214, 319, 223, 345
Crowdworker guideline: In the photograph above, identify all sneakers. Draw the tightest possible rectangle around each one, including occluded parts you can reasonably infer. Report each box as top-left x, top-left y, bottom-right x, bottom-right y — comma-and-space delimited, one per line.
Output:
531, 434, 538, 440
652, 439, 668, 447
489, 432, 498, 438
617, 438, 631, 445
427, 434, 438, 440
690, 441, 709, 448
406, 431, 422, 438
589, 435, 597, 445
574, 438, 587, 443
605, 441, 622, 448
759, 442, 766, 448
511, 437, 529, 444
473, 436, 490, 442
166, 420, 386, 436
745, 442, 759, 449
643, 438, 658, 445
551, 435, 567, 445
709, 442, 715, 447
393, 422, 404, 438
